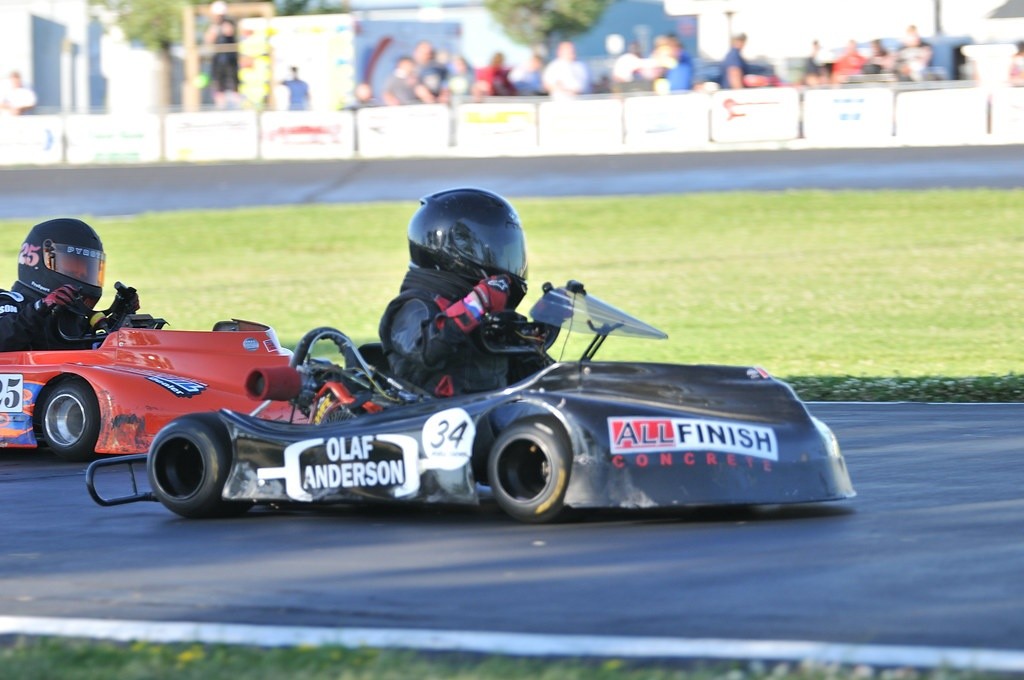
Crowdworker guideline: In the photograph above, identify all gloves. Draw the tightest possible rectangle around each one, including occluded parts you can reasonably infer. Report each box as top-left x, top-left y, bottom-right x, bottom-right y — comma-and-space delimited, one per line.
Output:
41, 284, 79, 311
115, 287, 140, 314
475, 275, 512, 314
530, 289, 574, 324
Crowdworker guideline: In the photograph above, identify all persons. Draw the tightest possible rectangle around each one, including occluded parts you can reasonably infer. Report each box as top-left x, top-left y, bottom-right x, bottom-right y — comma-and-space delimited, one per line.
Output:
383, 40, 515, 106
610, 31, 747, 91
347, 83, 379, 111
202, 2, 244, 112
0, 218, 139, 353
0, 72, 38, 115
800, 24, 936, 83
379, 188, 575, 400
280, 65, 312, 107
514, 42, 585, 96
1010, 44, 1024, 84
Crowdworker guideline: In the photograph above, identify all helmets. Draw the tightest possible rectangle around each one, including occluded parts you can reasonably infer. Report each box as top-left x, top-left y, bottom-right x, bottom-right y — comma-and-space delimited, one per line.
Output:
408, 187, 527, 310
18, 218, 106, 309
